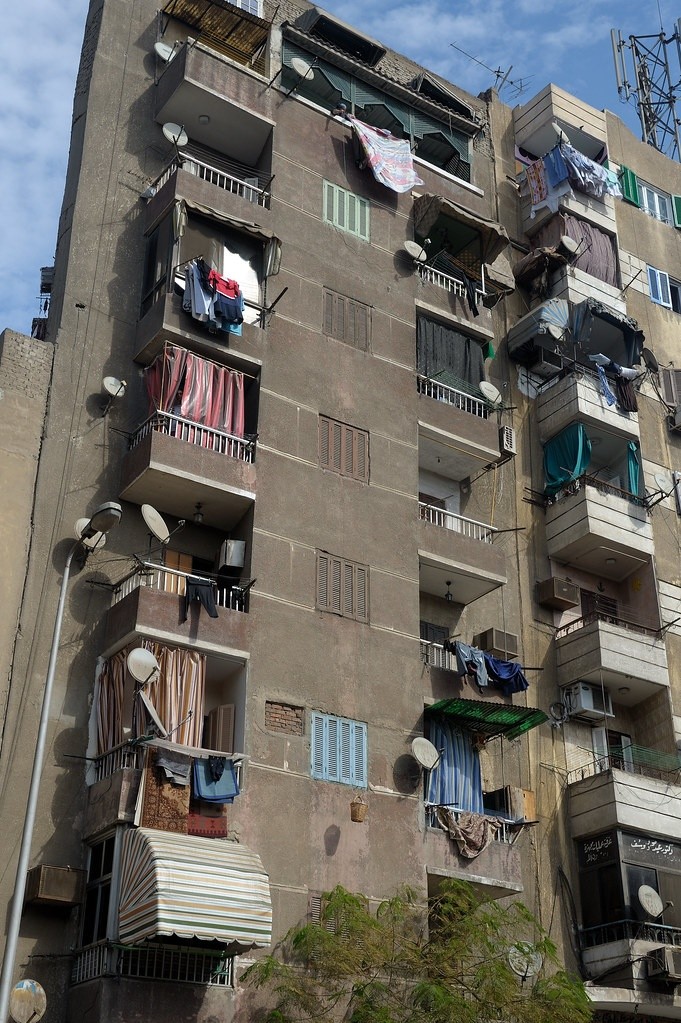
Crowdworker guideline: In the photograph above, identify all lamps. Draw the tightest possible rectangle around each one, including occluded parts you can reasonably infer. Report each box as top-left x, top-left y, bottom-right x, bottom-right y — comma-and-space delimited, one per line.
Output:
193, 502, 203, 526
444, 581, 453, 603
471, 725, 486, 751
441, 226, 452, 250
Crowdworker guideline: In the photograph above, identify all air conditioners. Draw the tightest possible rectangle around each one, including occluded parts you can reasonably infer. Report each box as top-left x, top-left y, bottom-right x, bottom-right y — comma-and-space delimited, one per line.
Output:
25, 864, 88, 910
565, 682, 615, 726
536, 577, 580, 612
528, 347, 564, 377
649, 946, 680, 983
218, 540, 245, 572
473, 628, 518, 660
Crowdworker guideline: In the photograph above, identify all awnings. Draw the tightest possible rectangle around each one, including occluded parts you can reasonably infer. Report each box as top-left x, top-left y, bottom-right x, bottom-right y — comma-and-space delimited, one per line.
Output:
414, 193, 516, 300
424, 697, 551, 742
574, 297, 646, 365
506, 299, 569, 354
295, 7, 387, 68
118, 827, 272, 957
